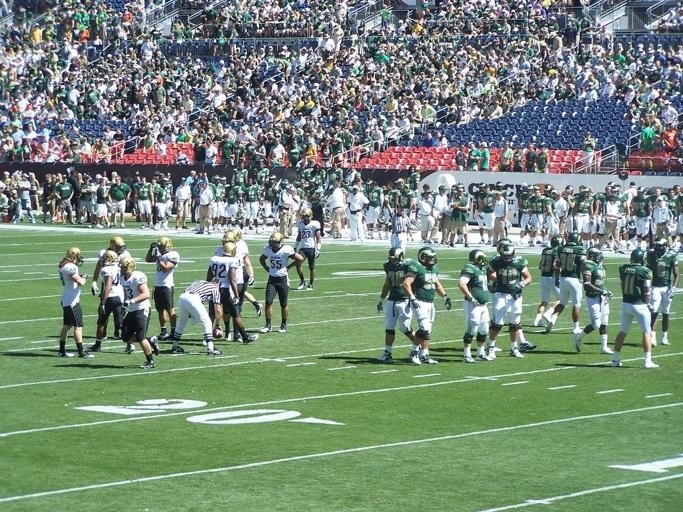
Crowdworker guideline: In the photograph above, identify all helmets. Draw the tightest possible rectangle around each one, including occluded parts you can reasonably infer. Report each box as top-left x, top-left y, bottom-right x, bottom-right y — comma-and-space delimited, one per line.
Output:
300, 209, 312, 221
497, 238, 515, 261
223, 229, 242, 257
65, 247, 81, 261
102, 252, 118, 267
417, 246, 437, 268
269, 232, 284, 249
630, 249, 645, 266
158, 237, 173, 252
585, 247, 603, 263
468, 249, 487, 267
108, 236, 125, 251
118, 257, 137, 275
388, 247, 403, 265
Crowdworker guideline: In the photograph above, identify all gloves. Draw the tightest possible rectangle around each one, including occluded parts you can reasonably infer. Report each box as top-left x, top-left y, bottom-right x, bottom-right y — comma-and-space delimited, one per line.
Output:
468, 296, 479, 306
247, 276, 255, 286
601, 289, 611, 303
98, 303, 106, 314
443, 296, 451, 312
232, 298, 240, 306
666, 288, 676, 299
410, 298, 420, 310
376, 298, 383, 313
510, 282, 524, 296
314, 248, 320, 259
122, 300, 134, 307
153, 249, 161, 263
90, 282, 99, 297
150, 240, 159, 249
648, 303, 655, 312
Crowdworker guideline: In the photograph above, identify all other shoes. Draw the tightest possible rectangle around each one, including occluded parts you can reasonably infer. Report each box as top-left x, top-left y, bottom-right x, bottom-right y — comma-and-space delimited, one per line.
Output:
332, 232, 498, 248
520, 235, 549, 248
582, 238, 648, 254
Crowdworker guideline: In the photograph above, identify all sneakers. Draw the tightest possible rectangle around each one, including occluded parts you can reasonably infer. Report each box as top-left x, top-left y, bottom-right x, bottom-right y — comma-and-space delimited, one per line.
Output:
643, 362, 659, 369
89, 325, 286, 357
573, 336, 582, 353
463, 347, 526, 364
78, 352, 95, 359
58, 351, 75, 358
382, 352, 394, 364
9, 218, 294, 239
256, 303, 264, 318
420, 355, 437, 365
297, 281, 314, 291
599, 348, 614, 355
534, 315, 583, 334
519, 340, 537, 352
660, 339, 669, 346
611, 360, 623, 367
410, 350, 420, 365
650, 340, 656, 347
140, 360, 156, 368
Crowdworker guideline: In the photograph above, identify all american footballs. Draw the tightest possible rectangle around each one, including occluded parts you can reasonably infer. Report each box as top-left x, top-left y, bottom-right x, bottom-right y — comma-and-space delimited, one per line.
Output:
214, 329, 222, 338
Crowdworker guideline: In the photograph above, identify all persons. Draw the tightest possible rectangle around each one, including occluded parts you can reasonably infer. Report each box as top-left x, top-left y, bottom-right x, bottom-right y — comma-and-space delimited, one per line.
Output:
376, 238, 683, 368
0, 1, 683, 258
59, 210, 323, 368
